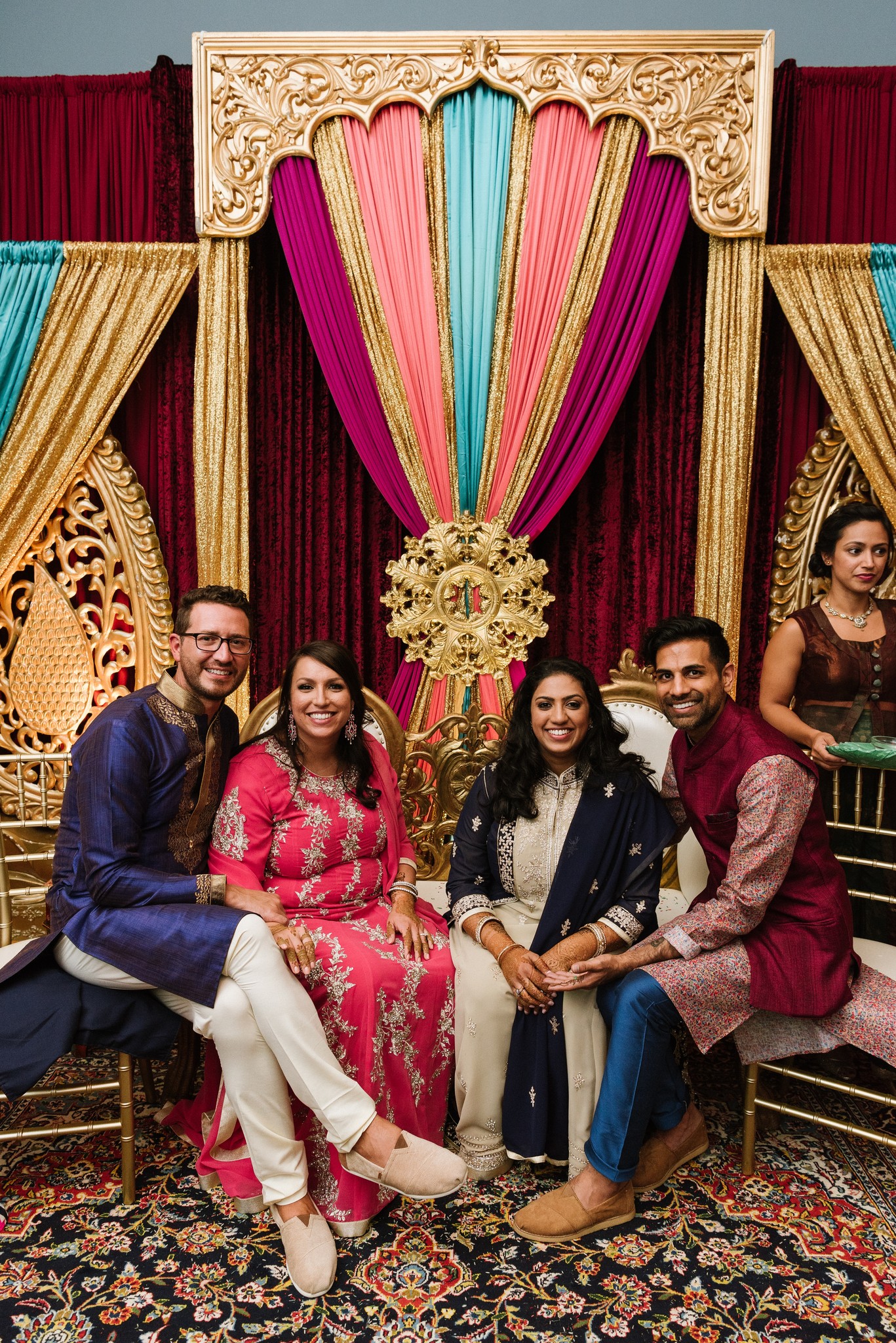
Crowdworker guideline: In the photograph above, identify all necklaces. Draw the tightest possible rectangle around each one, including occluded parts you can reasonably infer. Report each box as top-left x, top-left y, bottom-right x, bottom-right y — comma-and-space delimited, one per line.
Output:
824, 593, 874, 632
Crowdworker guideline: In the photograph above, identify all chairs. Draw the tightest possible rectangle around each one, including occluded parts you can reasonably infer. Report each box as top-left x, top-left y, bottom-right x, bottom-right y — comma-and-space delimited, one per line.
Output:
741, 747, 896, 1173
0, 755, 137, 1207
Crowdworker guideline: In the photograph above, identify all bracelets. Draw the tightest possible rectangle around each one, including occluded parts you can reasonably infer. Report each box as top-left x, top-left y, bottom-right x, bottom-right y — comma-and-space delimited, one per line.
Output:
387, 887, 418, 904
390, 881, 419, 897
578, 923, 607, 959
475, 915, 506, 950
497, 943, 525, 970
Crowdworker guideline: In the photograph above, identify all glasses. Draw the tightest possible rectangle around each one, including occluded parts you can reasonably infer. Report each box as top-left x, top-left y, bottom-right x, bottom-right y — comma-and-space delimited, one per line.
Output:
177, 632, 256, 655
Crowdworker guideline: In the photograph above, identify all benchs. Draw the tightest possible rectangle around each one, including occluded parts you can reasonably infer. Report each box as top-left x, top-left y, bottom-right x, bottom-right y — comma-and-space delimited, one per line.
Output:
235, 647, 699, 920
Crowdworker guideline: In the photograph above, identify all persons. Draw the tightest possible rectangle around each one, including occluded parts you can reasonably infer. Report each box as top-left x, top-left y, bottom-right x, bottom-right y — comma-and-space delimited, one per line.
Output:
755, 496, 895, 975
50, 579, 470, 1303
205, 634, 459, 1243
501, 610, 862, 1247
430, 652, 667, 1200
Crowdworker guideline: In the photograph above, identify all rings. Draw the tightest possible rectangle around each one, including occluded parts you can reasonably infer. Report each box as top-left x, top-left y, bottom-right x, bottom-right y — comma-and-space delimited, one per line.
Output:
419, 932, 428, 937
540, 990, 547, 995
574, 976, 577, 980
515, 987, 525, 997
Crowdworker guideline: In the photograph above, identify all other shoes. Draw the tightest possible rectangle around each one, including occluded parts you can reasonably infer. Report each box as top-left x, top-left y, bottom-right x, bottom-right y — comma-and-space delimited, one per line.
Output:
268, 1190, 338, 1298
461, 1149, 514, 1182
512, 1179, 636, 1242
338, 1127, 469, 1199
631, 1109, 710, 1194
